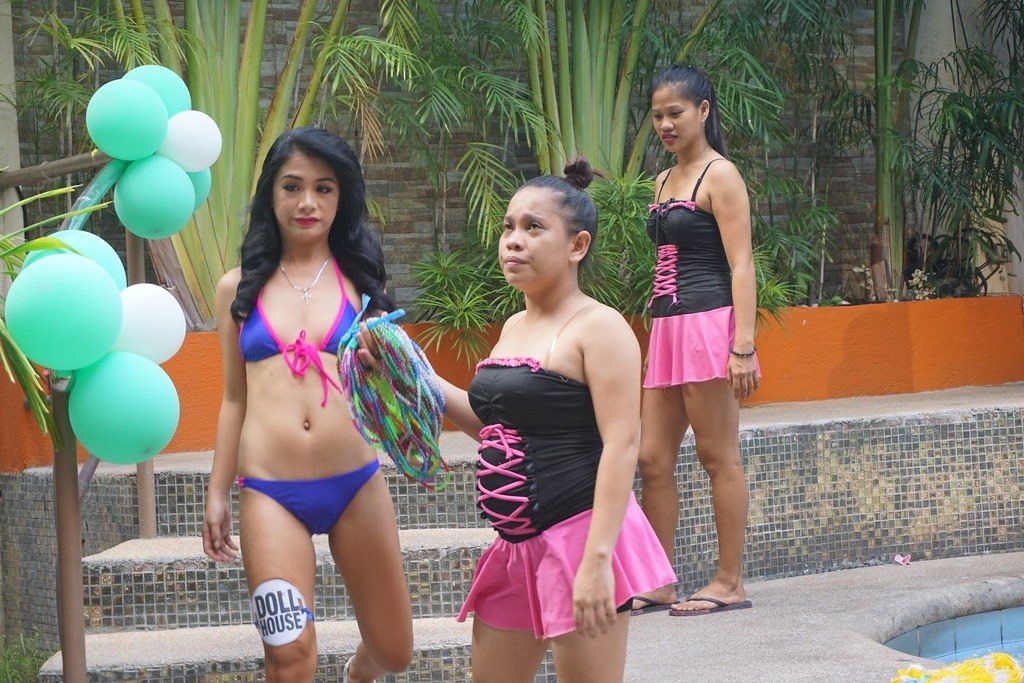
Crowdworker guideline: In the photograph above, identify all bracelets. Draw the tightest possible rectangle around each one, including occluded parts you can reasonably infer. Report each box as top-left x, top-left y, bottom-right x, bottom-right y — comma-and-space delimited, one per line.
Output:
729, 346, 757, 357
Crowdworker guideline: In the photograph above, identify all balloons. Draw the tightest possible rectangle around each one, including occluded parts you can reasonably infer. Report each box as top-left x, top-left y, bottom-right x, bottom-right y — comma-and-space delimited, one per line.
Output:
5, 230, 187, 464
86, 65, 223, 240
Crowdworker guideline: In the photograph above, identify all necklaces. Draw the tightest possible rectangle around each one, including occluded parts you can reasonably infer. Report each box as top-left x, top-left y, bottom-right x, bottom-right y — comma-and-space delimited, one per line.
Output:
279, 255, 331, 304
337, 314, 451, 492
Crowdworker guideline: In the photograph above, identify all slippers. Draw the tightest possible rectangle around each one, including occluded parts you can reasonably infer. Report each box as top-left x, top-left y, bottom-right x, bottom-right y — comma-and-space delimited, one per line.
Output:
669, 592, 752, 616
631, 595, 681, 616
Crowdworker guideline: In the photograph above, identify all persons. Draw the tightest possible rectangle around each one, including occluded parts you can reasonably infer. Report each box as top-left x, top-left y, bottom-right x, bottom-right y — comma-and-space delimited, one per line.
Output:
631, 63, 760, 618
358, 160, 677, 683
201, 127, 414, 683
